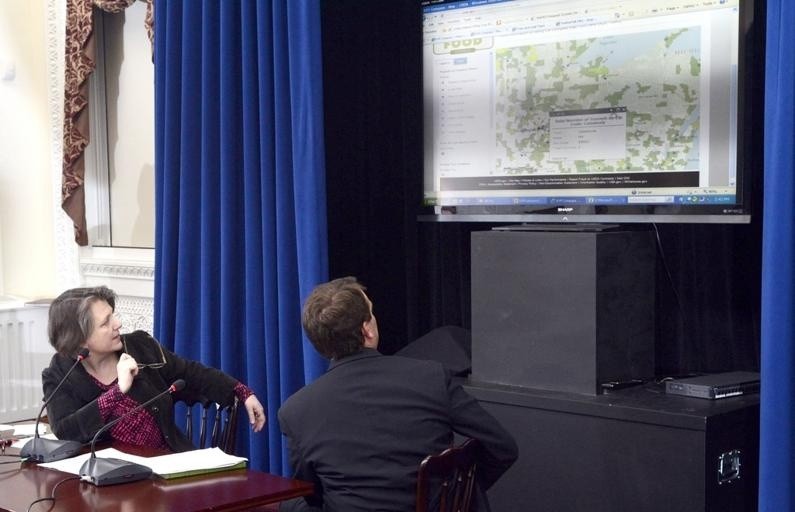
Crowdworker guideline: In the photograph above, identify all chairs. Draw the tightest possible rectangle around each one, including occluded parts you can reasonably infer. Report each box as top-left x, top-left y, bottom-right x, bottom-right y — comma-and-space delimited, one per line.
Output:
418, 437, 493, 512
172, 384, 241, 463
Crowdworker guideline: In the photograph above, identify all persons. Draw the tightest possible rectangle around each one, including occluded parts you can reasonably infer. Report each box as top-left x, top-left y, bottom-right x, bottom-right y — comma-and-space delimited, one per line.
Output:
278, 276, 519, 512
43, 287, 267, 452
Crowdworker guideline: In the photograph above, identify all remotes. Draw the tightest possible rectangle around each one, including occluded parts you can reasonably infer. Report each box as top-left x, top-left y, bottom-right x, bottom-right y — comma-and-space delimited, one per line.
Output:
602, 378, 643, 389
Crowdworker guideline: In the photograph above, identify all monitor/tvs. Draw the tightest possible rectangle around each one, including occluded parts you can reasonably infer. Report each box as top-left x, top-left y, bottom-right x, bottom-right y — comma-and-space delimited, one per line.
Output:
414, 0, 753, 225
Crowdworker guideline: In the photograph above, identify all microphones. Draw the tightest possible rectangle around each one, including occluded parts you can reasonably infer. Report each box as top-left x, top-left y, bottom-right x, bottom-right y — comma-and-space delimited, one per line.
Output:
78, 378, 186, 486
19, 347, 89, 463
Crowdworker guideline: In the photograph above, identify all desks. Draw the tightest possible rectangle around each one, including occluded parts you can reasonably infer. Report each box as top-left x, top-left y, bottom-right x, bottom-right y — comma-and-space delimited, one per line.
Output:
1, 413, 317, 511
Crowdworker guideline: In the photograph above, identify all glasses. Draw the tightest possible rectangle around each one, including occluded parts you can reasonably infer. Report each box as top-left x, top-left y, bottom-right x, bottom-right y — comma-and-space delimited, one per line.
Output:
123, 334, 167, 370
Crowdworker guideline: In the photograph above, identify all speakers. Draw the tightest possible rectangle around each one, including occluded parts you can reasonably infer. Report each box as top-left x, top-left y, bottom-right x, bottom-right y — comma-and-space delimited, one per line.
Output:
469, 228, 657, 396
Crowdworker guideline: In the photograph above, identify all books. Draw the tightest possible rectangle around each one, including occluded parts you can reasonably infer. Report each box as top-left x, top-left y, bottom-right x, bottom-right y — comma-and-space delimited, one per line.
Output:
149, 447, 247, 479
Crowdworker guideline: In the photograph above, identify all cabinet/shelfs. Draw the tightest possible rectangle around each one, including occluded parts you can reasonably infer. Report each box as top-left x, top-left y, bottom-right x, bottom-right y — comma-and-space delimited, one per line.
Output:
442, 230, 759, 512
